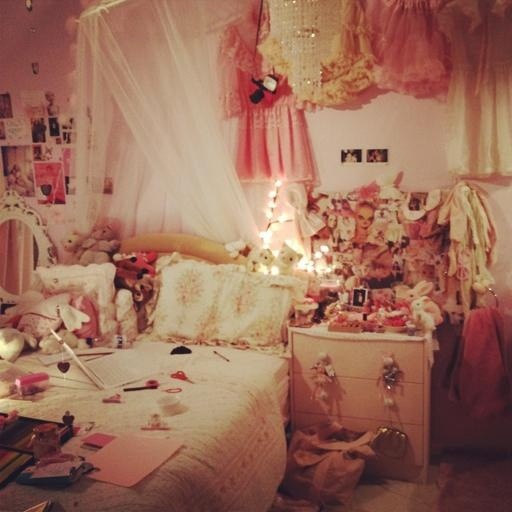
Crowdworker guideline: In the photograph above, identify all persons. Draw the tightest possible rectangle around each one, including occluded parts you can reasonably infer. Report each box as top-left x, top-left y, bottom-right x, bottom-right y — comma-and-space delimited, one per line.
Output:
44, 91, 60, 116
7, 163, 28, 196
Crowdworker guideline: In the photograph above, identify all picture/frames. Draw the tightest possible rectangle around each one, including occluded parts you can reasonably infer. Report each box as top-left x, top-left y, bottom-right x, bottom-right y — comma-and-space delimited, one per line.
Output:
348, 287, 369, 312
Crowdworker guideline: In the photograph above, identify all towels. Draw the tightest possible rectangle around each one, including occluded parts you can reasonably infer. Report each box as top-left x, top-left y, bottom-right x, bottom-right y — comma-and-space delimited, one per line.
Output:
448, 307, 512, 421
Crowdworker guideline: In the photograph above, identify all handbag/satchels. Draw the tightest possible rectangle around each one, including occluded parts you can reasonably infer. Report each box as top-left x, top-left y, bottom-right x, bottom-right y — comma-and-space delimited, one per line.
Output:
375, 426, 407, 457
277, 423, 376, 510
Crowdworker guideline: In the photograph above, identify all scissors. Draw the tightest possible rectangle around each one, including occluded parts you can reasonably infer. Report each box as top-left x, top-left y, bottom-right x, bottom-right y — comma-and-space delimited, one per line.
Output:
171, 371, 194, 384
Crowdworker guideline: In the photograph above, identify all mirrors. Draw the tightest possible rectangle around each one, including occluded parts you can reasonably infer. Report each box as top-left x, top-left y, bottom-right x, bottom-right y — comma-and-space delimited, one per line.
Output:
0, 189, 50, 303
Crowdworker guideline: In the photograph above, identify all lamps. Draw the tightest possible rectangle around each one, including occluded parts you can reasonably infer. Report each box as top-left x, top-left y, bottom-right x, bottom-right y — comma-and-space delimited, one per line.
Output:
249, 73, 279, 104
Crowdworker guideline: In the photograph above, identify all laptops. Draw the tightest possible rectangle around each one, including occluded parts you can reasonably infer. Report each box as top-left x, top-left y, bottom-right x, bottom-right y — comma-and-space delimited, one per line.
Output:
48, 326, 164, 390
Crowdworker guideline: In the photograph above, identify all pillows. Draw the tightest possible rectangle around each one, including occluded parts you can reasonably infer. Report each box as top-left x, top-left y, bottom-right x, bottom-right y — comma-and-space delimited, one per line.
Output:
37, 251, 308, 354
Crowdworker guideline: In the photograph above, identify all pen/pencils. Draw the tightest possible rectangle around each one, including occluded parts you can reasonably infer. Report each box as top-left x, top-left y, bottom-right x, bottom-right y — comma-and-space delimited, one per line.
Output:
123, 386, 157, 392
213, 351, 229, 361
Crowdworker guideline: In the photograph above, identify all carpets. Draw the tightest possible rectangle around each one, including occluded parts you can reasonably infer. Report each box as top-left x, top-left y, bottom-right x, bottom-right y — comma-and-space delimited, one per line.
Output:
317, 463, 453, 512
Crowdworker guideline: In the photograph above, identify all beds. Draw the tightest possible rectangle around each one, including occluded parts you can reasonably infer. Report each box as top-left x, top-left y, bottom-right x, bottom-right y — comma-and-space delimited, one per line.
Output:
0, 233, 310, 512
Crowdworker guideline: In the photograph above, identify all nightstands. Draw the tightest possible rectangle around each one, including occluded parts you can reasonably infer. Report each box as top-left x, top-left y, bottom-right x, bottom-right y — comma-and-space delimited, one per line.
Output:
288, 313, 434, 484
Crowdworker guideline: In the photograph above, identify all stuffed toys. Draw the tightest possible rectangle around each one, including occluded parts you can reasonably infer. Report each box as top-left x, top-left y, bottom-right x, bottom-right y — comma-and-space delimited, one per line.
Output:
0, 217, 158, 363
260, 242, 445, 336
